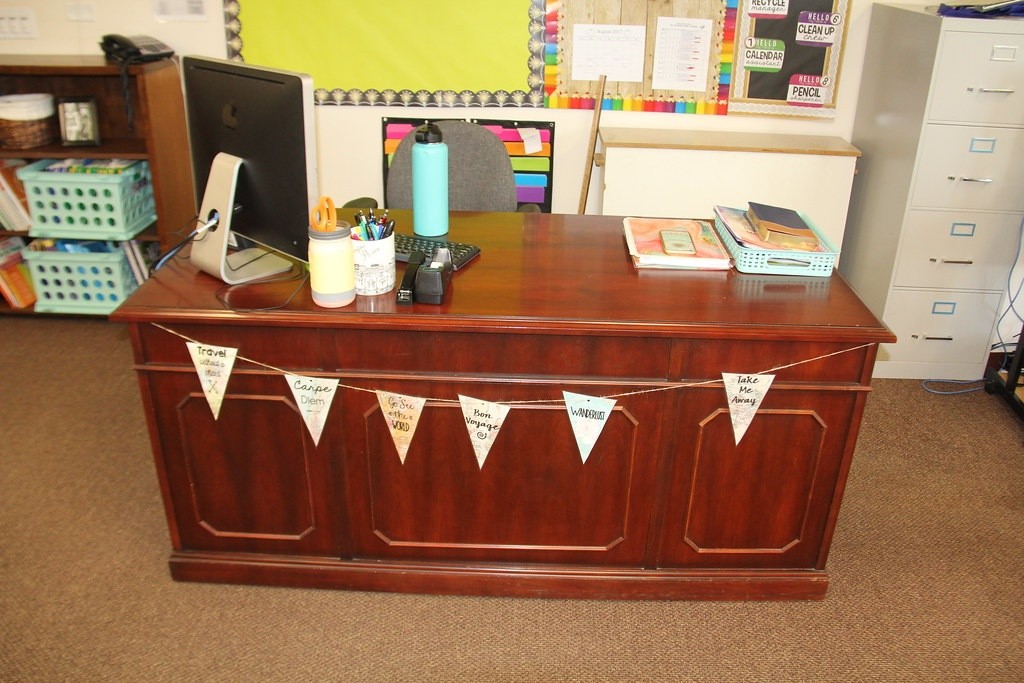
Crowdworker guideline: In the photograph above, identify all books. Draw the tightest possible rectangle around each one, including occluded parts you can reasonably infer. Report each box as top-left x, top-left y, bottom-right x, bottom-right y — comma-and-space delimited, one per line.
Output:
0, 159, 143, 232
621, 202, 821, 271
0, 235, 160, 310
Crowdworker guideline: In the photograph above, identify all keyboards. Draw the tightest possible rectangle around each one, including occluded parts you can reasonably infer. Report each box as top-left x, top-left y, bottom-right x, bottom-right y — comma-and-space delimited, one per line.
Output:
394, 234, 480, 274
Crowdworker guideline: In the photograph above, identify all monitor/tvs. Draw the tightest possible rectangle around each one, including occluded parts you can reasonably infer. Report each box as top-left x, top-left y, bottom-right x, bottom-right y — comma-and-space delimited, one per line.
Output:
182, 54, 316, 286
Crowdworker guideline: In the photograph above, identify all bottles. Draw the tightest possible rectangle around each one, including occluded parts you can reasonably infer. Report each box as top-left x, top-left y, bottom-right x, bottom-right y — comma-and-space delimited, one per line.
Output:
411, 124, 448, 236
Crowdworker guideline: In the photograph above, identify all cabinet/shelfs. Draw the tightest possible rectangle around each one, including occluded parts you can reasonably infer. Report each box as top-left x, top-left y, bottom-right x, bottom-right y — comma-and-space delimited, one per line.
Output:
837, 0, 1024, 382
0, 52, 198, 319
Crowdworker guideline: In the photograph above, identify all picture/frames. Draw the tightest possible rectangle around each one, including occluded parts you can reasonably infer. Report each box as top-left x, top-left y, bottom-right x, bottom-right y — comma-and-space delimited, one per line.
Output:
56, 96, 102, 148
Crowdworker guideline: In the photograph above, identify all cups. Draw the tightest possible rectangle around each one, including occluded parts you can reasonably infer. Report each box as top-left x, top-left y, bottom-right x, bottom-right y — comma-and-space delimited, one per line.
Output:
351, 226, 396, 295
309, 220, 355, 308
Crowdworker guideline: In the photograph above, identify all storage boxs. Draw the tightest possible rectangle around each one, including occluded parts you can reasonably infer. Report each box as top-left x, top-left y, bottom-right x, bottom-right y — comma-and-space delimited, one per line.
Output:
712, 208, 843, 278
20, 241, 140, 315
14, 158, 157, 242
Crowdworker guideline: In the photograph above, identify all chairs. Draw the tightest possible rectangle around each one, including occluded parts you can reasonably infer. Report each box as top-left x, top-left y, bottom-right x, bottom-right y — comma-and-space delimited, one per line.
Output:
387, 119, 521, 212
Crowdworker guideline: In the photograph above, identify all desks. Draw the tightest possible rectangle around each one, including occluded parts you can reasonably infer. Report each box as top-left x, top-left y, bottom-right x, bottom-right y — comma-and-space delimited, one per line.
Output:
108, 207, 900, 604
598, 126, 863, 270
